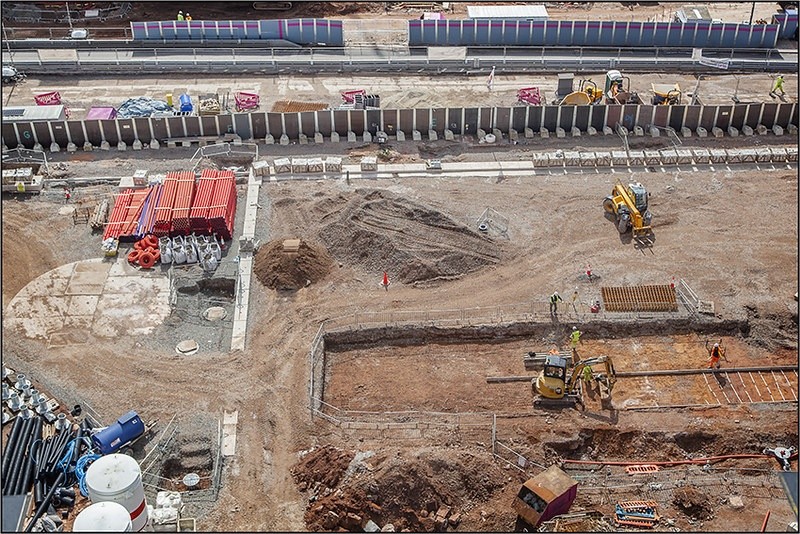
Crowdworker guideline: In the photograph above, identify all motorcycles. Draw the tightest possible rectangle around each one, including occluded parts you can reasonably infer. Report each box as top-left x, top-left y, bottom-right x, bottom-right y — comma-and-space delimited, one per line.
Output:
603, 178, 655, 245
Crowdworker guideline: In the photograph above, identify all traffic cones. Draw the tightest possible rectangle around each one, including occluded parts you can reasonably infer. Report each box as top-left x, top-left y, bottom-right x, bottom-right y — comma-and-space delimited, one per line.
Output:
64, 188, 71, 198
381, 272, 390, 286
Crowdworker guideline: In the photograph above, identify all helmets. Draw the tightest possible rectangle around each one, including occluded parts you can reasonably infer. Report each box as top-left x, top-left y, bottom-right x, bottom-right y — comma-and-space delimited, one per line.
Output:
554, 292, 558, 296
714, 343, 718, 347
573, 326, 577, 330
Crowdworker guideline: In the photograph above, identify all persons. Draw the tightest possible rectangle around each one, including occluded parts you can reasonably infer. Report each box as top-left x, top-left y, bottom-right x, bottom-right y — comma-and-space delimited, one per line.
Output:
707, 342, 725, 369
583, 365, 591, 386
772, 75, 787, 95
569, 325, 583, 351
549, 291, 563, 317
185, 12, 192, 20
611, 82, 618, 96
177, 10, 185, 20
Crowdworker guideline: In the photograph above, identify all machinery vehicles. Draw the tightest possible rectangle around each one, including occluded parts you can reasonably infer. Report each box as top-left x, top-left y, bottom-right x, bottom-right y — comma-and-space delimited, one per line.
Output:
651, 82, 682, 105
603, 69, 644, 104
559, 76, 605, 106
529, 351, 617, 410
